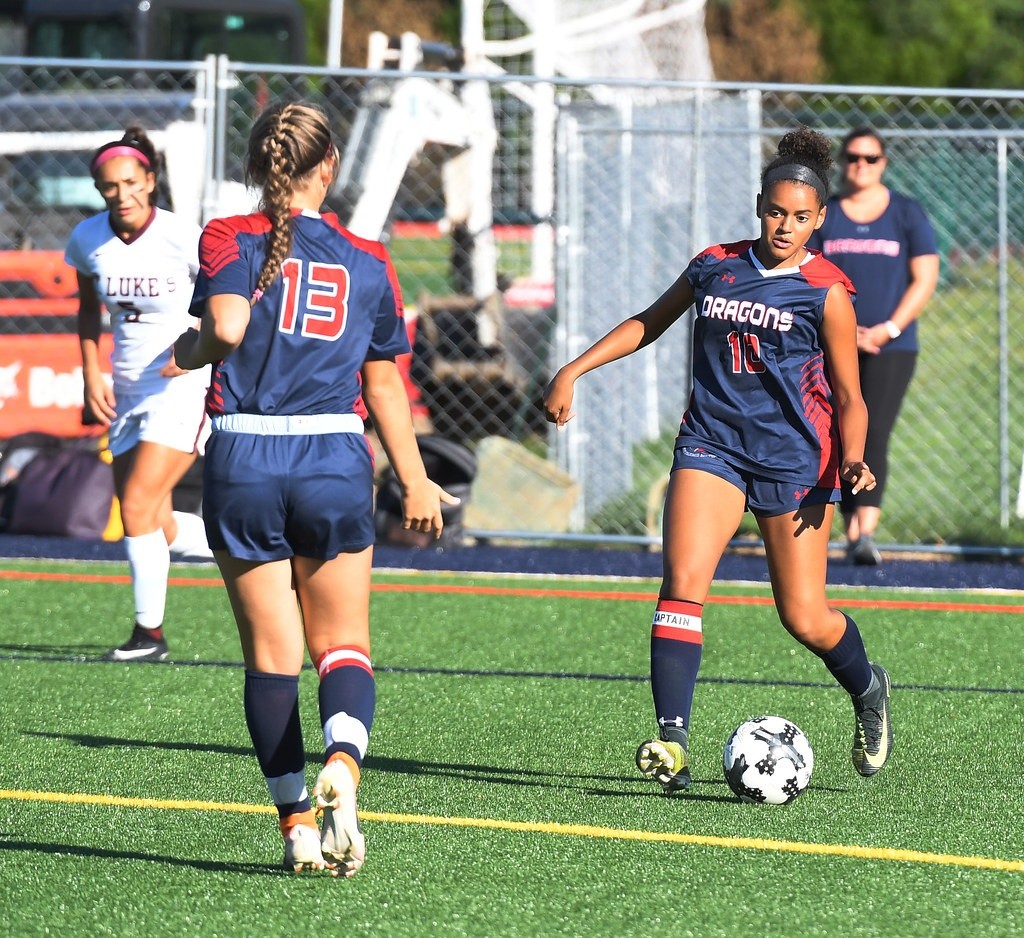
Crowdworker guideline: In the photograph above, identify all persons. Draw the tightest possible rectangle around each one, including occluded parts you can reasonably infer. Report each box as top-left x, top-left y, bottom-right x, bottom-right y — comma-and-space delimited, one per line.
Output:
66, 128, 214, 665
804, 128, 939, 566
543, 127, 895, 793
173, 105, 465, 876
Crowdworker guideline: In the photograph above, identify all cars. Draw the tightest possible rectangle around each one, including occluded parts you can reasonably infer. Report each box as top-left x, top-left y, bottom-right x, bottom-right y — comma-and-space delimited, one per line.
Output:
0, 84, 200, 463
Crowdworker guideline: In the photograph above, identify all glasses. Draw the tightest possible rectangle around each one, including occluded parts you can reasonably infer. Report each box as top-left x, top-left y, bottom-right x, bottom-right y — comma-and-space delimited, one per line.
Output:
845, 152, 880, 164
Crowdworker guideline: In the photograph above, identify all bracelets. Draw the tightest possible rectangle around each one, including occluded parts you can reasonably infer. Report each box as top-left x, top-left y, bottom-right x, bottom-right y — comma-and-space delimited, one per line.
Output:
884, 320, 901, 339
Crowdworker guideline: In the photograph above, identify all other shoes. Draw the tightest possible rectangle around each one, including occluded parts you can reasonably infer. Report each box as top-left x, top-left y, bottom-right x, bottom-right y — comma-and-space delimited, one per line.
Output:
845, 537, 883, 566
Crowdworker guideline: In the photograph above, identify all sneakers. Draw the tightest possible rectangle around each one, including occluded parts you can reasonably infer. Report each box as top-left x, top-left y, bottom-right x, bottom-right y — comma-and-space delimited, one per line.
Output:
636, 739, 691, 789
312, 758, 366, 878
850, 665, 893, 776
282, 825, 323, 872
108, 624, 169, 662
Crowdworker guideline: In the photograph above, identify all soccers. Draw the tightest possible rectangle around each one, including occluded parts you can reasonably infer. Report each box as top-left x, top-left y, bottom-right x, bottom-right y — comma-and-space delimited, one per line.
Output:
723, 716, 815, 806
